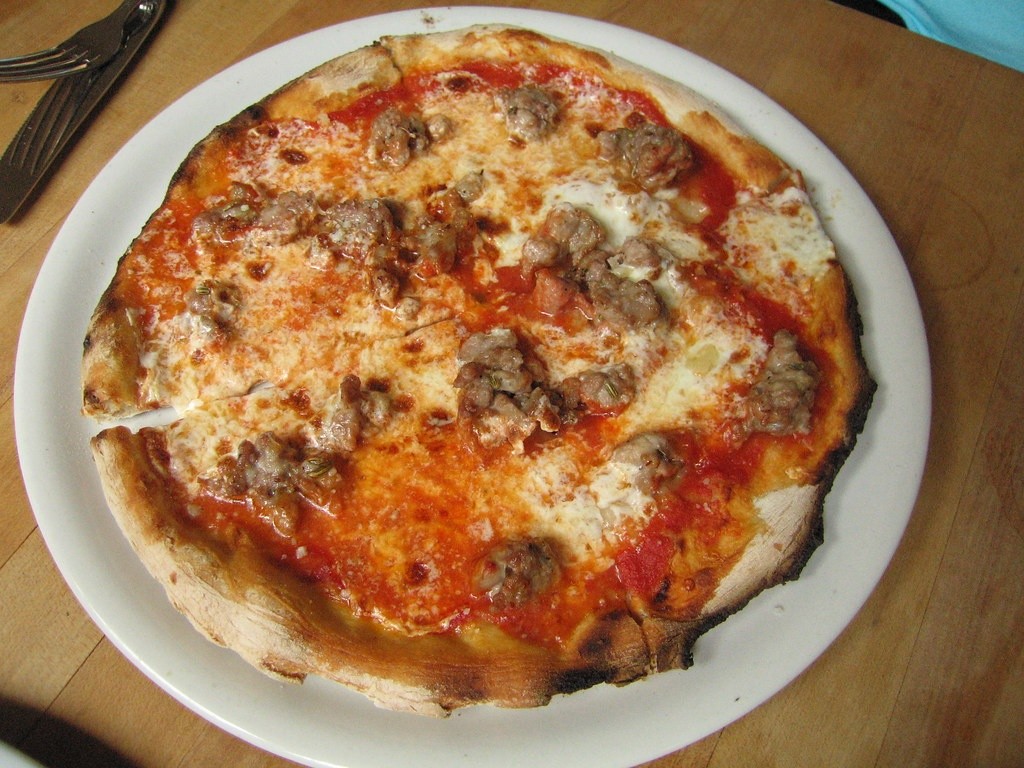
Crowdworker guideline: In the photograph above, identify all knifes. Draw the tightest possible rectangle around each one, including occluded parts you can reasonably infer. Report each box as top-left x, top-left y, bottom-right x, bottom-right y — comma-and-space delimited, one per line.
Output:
0, 0, 169, 224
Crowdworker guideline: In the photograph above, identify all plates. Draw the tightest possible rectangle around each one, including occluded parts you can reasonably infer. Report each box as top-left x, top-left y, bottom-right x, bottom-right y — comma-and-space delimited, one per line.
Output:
14, 7, 931, 768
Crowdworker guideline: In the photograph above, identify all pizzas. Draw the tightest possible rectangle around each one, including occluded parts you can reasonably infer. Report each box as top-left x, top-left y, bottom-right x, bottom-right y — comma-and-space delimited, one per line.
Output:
78, 19, 879, 719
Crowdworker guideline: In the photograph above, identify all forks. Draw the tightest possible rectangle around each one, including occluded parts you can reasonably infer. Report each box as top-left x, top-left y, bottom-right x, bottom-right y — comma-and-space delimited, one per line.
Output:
0, 0, 144, 83
9, 10, 147, 175
